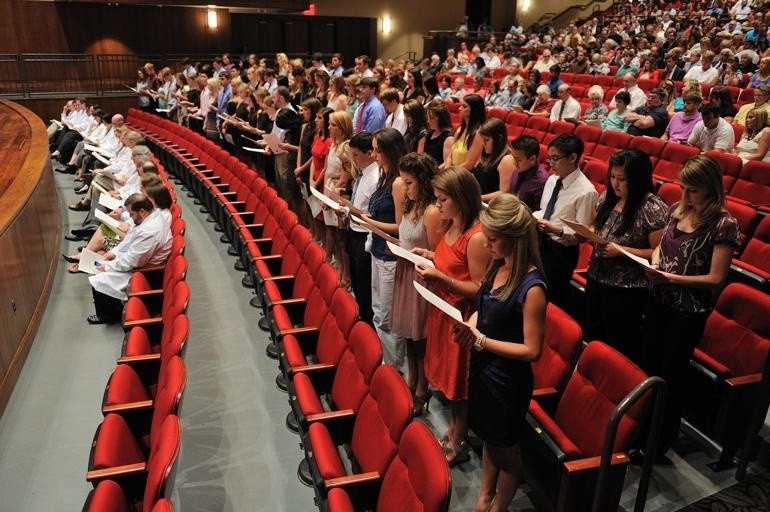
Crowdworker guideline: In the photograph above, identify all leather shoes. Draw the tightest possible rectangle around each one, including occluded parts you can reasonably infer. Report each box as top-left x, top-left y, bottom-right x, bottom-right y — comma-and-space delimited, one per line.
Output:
73, 175, 89, 194
88, 316, 104, 323
629, 449, 647, 463
63, 225, 99, 272
69, 203, 90, 210
51, 153, 76, 175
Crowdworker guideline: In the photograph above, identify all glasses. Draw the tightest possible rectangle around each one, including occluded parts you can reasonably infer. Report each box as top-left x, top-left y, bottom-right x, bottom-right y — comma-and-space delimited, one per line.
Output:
544, 156, 567, 163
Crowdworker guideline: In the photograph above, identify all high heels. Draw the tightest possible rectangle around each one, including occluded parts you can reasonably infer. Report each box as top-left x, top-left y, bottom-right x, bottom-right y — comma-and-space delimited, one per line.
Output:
410, 384, 433, 418
439, 434, 468, 466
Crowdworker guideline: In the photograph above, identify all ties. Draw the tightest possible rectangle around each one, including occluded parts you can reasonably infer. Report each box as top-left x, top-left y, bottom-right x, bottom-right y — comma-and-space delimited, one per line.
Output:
543, 179, 561, 222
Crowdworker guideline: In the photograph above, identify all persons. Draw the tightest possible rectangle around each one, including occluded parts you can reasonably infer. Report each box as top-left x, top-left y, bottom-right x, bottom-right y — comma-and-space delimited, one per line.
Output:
47, 97, 173, 326
137, 2, 770, 511
